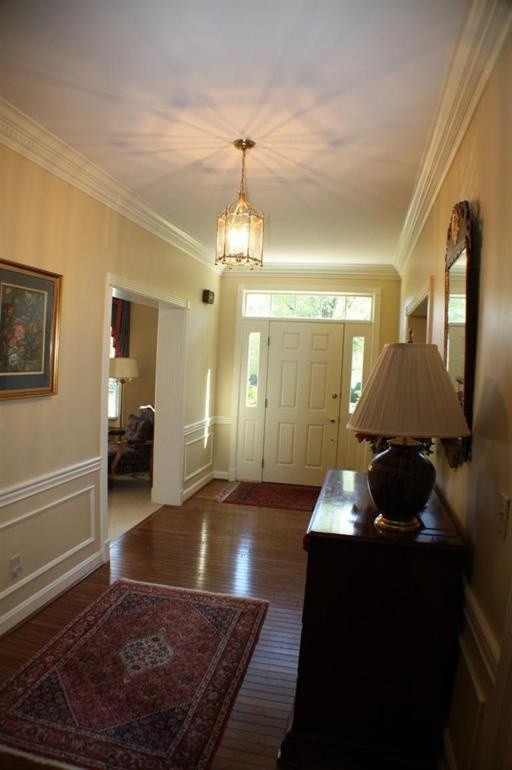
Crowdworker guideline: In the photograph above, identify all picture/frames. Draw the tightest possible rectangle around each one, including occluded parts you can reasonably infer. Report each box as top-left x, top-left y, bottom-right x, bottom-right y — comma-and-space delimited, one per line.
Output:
0, 256, 65, 400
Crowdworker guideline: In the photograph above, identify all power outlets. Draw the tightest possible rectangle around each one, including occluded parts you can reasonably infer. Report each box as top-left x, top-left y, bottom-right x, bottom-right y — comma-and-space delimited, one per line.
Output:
486, 491, 509, 538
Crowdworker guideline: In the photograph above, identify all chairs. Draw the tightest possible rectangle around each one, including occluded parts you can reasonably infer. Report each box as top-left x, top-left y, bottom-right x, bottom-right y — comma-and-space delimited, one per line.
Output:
106, 404, 155, 494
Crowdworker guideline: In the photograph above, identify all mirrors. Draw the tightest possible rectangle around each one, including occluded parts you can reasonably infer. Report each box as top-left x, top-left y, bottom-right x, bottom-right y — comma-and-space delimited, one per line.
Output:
430, 195, 481, 473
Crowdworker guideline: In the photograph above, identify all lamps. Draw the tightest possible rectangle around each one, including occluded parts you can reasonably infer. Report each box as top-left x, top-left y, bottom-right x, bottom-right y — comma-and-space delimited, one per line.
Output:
209, 132, 269, 276
346, 341, 469, 532
109, 354, 141, 442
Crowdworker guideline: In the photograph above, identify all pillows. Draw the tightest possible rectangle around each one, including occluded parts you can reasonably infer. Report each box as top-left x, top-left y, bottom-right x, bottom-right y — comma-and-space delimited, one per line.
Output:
123, 413, 146, 444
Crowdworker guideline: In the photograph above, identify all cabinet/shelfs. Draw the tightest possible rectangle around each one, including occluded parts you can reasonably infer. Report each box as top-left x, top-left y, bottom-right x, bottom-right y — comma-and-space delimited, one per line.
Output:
274, 466, 473, 769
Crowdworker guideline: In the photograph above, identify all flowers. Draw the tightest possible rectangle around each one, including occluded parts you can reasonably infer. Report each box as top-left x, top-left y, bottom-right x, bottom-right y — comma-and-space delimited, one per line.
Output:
354, 431, 435, 457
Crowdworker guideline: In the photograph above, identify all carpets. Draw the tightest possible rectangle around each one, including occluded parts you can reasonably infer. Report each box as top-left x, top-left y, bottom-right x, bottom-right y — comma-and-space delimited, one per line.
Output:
214, 478, 321, 514
0, 571, 269, 765
